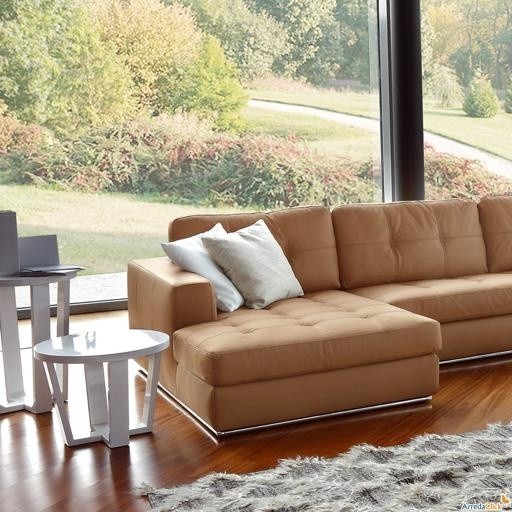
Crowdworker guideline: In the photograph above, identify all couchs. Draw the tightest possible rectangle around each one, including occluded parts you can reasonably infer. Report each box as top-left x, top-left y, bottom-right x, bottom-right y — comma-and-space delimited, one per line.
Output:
124, 195, 511, 438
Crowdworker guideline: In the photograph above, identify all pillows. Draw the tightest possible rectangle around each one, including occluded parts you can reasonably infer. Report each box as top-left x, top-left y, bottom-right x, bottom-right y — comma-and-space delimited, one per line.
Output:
158, 213, 305, 315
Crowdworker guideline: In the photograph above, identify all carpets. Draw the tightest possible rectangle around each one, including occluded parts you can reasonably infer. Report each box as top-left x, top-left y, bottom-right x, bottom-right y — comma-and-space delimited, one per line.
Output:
138, 421, 511, 511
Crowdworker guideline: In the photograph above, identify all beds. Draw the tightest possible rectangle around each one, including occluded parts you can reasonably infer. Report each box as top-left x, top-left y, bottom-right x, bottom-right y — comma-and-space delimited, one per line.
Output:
32, 329, 172, 452
